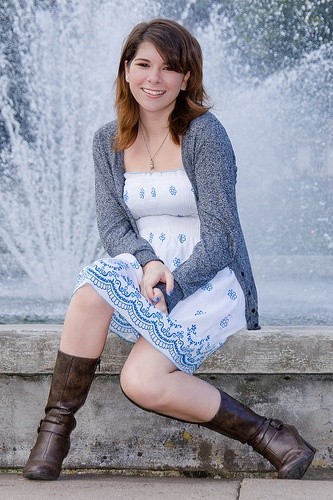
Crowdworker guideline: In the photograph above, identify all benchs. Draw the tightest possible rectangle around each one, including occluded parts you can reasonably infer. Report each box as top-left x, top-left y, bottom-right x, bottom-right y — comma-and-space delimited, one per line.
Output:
0, 324, 332, 472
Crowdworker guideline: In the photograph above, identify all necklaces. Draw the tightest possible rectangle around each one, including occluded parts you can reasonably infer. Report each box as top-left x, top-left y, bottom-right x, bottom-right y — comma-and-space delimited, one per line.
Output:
139, 122, 169, 169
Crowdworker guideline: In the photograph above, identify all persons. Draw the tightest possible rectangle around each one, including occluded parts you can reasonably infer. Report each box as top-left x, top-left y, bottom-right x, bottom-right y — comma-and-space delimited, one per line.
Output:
24, 20, 317, 479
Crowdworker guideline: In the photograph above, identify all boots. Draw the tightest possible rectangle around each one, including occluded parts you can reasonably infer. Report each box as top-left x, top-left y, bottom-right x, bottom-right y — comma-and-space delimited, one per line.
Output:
22, 349, 102, 481
197, 386, 316, 479
